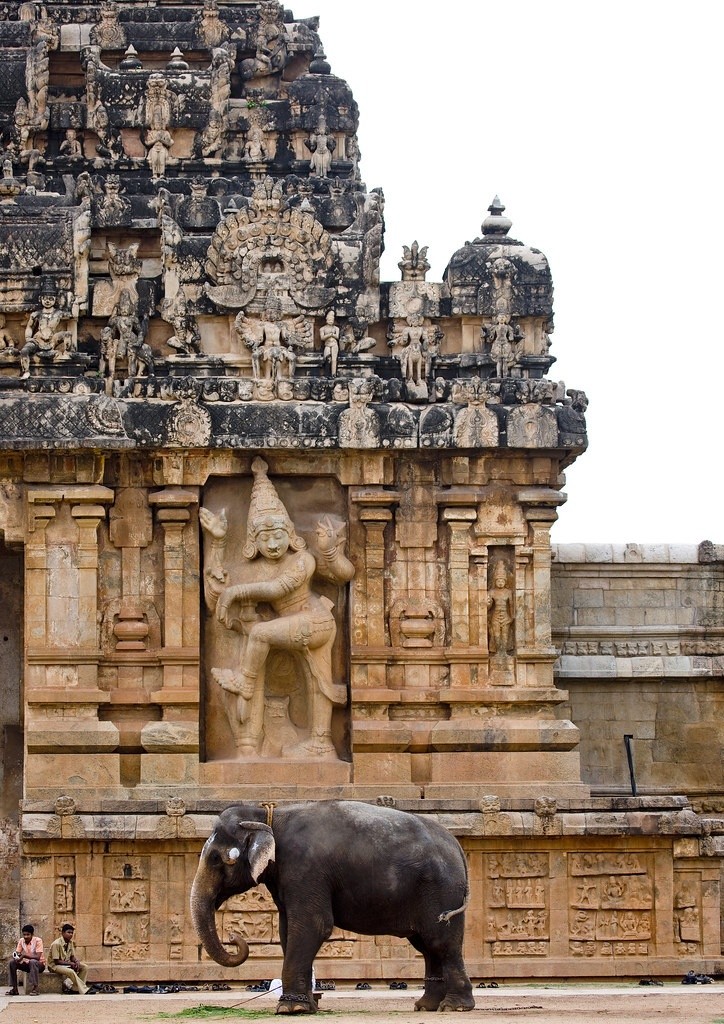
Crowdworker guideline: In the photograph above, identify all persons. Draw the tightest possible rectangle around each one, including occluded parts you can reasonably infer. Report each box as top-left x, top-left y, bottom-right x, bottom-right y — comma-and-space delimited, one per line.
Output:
47, 923, 98, 995
1, 0, 529, 399
198, 459, 353, 768
4, 924, 48, 996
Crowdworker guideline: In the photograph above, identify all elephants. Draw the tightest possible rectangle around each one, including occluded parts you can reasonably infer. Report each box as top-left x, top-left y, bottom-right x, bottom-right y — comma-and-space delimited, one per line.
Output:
190, 798, 474, 1015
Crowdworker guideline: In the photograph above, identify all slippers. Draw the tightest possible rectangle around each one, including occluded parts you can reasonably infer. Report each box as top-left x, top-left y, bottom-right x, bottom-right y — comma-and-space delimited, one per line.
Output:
30, 989, 40, 995
96, 981, 231, 995
246, 979, 270, 992
476, 982, 486, 988
355, 982, 370, 990
487, 982, 499, 988
5, 989, 19, 995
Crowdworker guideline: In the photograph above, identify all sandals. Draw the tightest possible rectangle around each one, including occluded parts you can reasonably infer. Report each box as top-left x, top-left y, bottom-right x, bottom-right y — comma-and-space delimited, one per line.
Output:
390, 982, 407, 989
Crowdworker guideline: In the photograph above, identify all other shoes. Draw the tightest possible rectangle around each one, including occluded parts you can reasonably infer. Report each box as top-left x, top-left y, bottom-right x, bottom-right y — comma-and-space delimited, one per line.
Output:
682, 970, 714, 985
86, 988, 96, 994
315, 980, 335, 991
639, 978, 663, 986
67, 988, 78, 994
